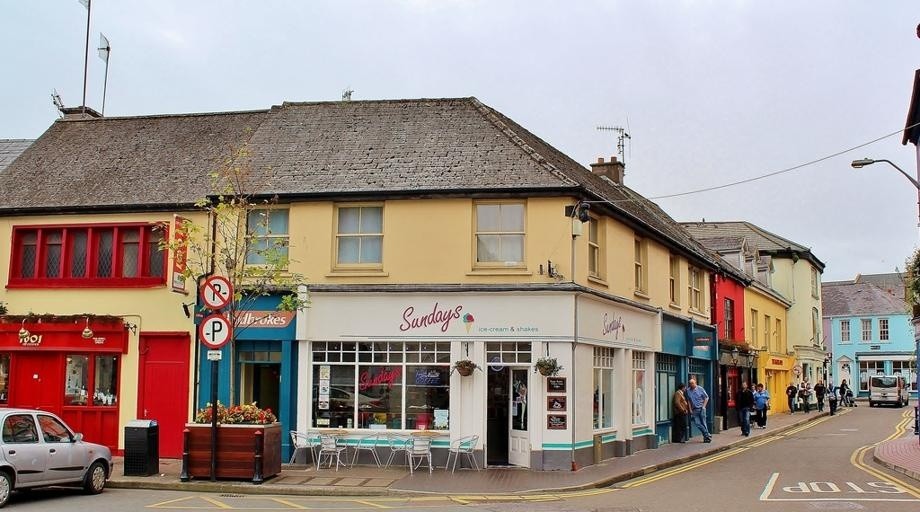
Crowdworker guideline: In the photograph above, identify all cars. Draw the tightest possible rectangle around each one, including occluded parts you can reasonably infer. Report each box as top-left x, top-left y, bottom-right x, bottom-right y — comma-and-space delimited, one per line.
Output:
0, 407, 113, 509
312, 383, 391, 429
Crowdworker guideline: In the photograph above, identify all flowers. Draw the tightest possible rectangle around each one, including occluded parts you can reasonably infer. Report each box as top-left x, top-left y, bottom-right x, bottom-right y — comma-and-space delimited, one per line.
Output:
193, 398, 276, 424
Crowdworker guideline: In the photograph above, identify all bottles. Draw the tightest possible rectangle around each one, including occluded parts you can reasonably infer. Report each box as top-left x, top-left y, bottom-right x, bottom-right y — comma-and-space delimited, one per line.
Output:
346, 415, 352, 428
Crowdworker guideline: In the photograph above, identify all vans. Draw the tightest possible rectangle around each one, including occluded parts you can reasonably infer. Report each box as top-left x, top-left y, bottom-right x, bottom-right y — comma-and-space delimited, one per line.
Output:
868, 374, 910, 408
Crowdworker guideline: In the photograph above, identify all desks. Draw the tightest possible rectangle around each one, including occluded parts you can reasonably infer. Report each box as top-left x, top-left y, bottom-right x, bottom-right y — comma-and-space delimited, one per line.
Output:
411, 431, 440, 470
320, 429, 349, 466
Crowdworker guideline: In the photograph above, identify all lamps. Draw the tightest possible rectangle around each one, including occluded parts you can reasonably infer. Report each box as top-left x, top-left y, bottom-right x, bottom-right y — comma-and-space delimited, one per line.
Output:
731, 346, 739, 366
18, 317, 30, 338
747, 351, 755, 368
82, 317, 93, 338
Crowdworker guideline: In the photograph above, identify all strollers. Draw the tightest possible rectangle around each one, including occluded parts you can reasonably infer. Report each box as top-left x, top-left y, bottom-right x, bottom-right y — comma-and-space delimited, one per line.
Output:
840, 387, 857, 407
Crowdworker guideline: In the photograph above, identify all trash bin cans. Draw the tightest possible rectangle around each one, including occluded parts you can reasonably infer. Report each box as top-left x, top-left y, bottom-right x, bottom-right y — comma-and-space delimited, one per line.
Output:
124, 420, 159, 476
714, 416, 723, 434
829, 396, 837, 412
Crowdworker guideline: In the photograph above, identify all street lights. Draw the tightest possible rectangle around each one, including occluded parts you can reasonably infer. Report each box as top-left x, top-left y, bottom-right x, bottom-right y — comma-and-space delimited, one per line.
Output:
851, 136, 920, 439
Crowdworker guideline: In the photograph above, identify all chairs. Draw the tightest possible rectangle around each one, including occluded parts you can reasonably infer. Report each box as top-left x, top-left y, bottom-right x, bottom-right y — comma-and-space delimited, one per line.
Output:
404, 437, 432, 474
288, 430, 318, 467
352, 432, 381, 467
384, 430, 416, 468
445, 435, 480, 473
316, 434, 347, 471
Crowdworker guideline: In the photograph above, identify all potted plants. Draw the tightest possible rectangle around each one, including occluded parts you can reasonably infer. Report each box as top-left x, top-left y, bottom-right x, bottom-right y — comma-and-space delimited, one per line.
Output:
534, 357, 562, 376
448, 360, 486, 376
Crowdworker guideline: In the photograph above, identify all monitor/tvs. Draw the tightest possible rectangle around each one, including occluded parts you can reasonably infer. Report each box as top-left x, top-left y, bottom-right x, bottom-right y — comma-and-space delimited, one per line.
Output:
414, 368, 441, 386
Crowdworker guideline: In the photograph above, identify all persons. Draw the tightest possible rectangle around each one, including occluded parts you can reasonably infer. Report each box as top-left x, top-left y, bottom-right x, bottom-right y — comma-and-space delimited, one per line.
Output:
754, 383, 770, 429
685, 379, 712, 442
673, 383, 689, 443
736, 381, 754, 436
786, 376, 850, 415
750, 383, 758, 428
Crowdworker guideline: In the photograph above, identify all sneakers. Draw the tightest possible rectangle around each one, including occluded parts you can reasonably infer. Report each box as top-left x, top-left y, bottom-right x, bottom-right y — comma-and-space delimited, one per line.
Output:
703, 436, 712, 443
754, 425, 766, 429
741, 432, 749, 437
791, 411, 837, 416
